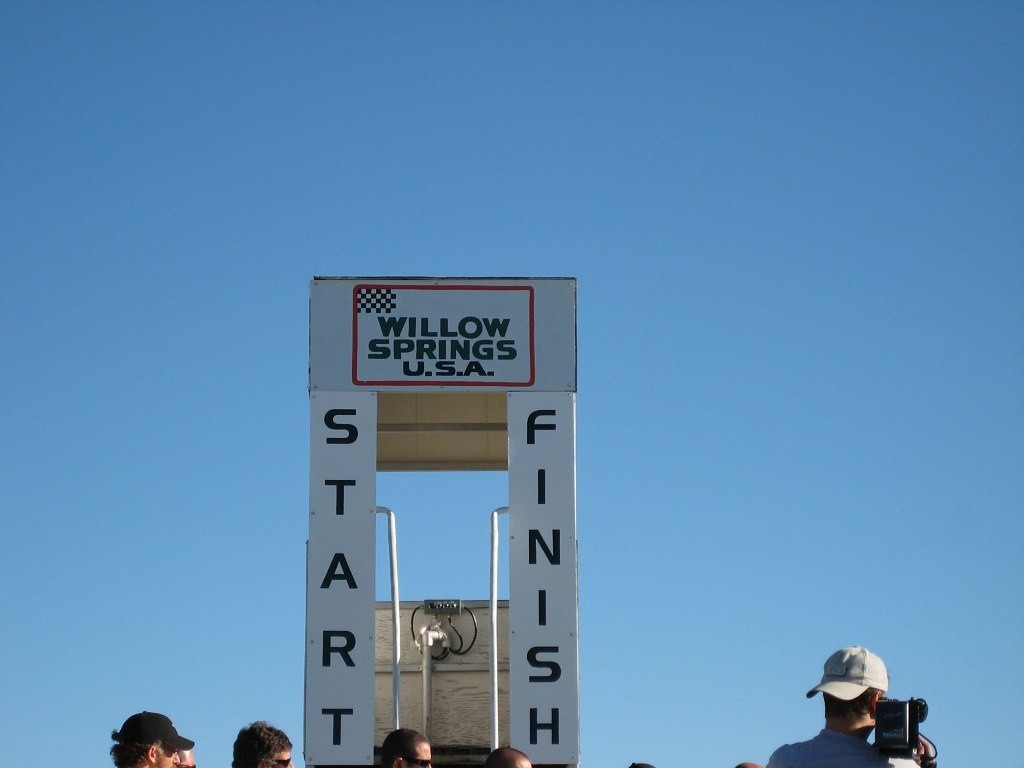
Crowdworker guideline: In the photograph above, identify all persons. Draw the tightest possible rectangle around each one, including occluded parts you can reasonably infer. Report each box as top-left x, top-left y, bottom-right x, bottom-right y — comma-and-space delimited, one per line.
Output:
380, 729, 431, 768
484, 747, 532, 768
735, 762, 765, 768
765, 645, 937, 768
232, 721, 294, 768
629, 763, 656, 768
109, 711, 196, 768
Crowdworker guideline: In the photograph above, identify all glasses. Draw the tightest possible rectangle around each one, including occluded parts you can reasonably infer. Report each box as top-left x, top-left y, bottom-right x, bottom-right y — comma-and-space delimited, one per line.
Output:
154, 745, 179, 757
393, 755, 431, 767
176, 764, 196, 768
272, 757, 290, 767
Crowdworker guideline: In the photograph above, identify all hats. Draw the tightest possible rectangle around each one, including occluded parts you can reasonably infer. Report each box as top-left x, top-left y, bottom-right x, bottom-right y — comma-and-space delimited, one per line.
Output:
119, 711, 195, 750
806, 646, 889, 700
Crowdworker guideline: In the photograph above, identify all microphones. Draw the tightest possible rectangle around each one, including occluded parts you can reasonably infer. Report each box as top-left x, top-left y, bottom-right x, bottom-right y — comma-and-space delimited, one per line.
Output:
917, 698, 929, 722
